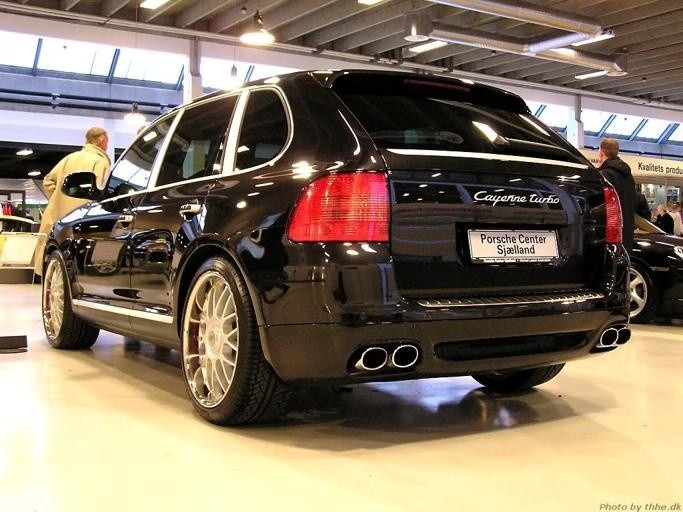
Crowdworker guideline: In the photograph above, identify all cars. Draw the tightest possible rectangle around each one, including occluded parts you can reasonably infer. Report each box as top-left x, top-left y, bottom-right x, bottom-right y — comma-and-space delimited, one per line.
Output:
0, 214, 40, 267
612, 207, 683, 329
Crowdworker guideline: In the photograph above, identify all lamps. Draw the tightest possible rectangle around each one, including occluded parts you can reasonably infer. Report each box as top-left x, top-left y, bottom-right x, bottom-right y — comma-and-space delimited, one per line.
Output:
404, 4, 431, 42
238, 9, 275, 47
16, 148, 34, 156
27, 170, 42, 176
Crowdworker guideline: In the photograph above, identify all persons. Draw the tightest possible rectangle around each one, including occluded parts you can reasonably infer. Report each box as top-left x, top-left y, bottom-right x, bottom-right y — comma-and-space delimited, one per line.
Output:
30, 127, 112, 281
668, 203, 683, 236
653, 203, 674, 235
633, 184, 650, 222
595, 138, 637, 254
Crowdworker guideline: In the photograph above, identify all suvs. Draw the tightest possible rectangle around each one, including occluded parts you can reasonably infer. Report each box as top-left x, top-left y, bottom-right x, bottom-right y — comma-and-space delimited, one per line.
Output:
38, 65, 636, 426
